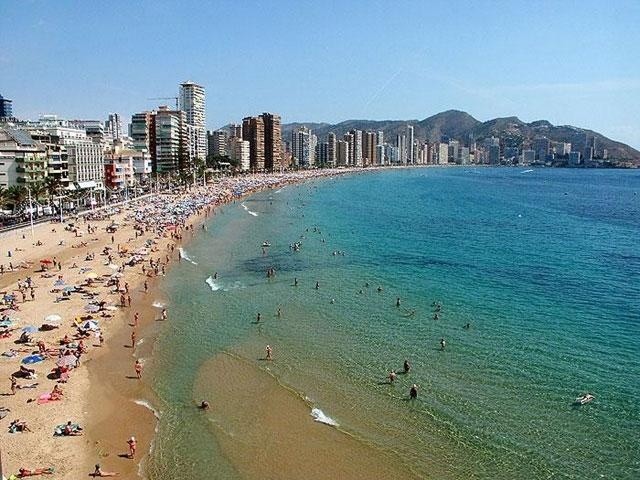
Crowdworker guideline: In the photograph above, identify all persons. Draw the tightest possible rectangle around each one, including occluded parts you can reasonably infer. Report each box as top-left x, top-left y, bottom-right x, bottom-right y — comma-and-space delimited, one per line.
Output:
0, 169, 472, 480
574, 393, 596, 406
